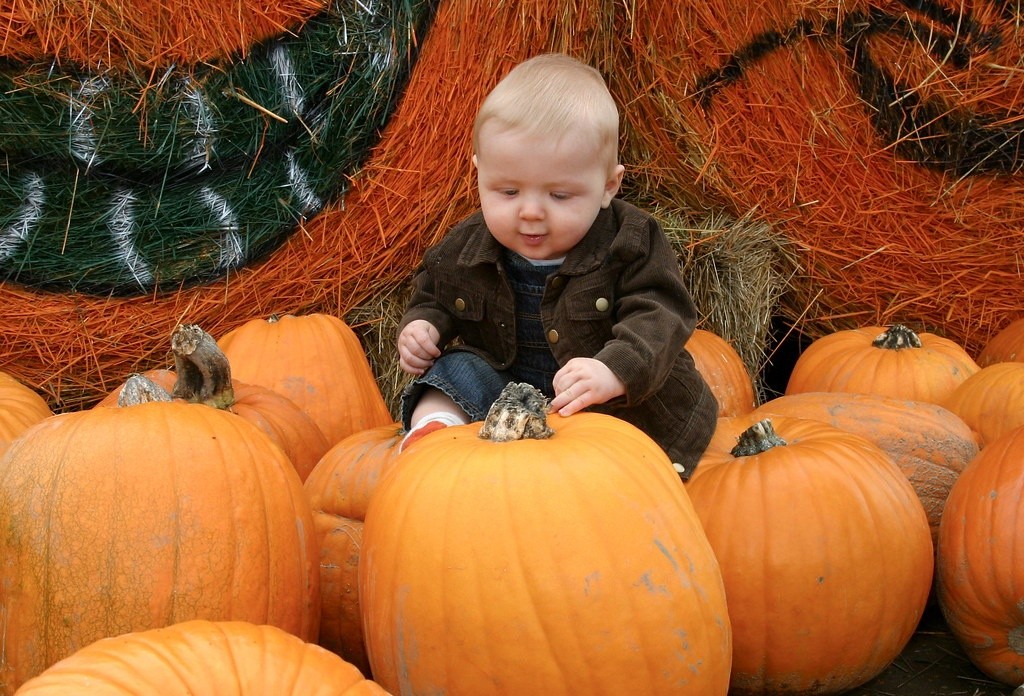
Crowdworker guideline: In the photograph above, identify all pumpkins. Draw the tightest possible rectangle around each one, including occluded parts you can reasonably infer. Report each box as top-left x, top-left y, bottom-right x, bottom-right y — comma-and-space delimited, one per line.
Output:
1, 315, 1024, 696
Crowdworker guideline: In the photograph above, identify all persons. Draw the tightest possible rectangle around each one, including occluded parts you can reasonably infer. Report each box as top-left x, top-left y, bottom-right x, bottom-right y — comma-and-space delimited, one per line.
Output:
396, 54, 720, 485
